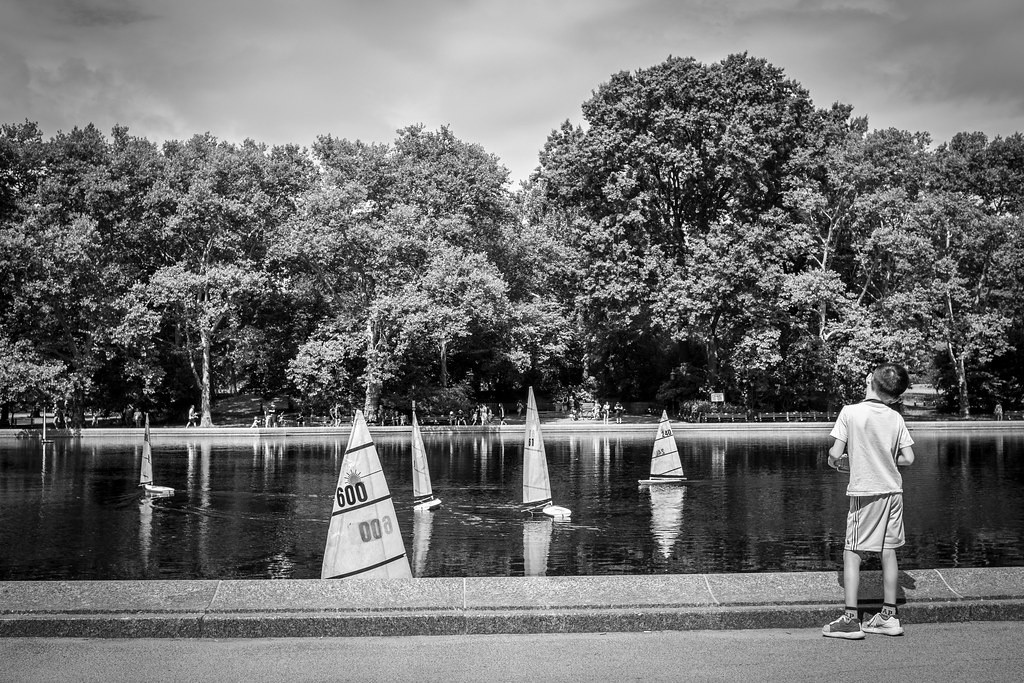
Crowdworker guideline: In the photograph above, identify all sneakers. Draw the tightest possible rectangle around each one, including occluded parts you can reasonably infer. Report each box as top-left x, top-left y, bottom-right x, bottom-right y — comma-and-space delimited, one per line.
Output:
861, 612, 904, 636
822, 616, 865, 639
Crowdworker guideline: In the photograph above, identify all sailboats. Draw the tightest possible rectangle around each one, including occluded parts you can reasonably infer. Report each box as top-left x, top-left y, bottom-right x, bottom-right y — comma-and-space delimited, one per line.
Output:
637, 410, 689, 486
409, 398, 442, 509
320, 408, 417, 579
455, 386, 571, 518
138, 413, 176, 507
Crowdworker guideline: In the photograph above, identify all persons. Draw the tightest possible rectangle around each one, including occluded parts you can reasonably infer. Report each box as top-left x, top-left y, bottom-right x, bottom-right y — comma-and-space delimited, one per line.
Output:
53, 405, 143, 428
185, 405, 198, 427
820, 363, 915, 640
995, 404, 1003, 421
250, 389, 624, 428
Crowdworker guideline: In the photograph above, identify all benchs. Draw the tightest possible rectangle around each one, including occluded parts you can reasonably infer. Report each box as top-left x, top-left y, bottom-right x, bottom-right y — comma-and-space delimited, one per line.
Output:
704, 412, 748, 424
261, 414, 545, 427
758, 412, 839, 422
